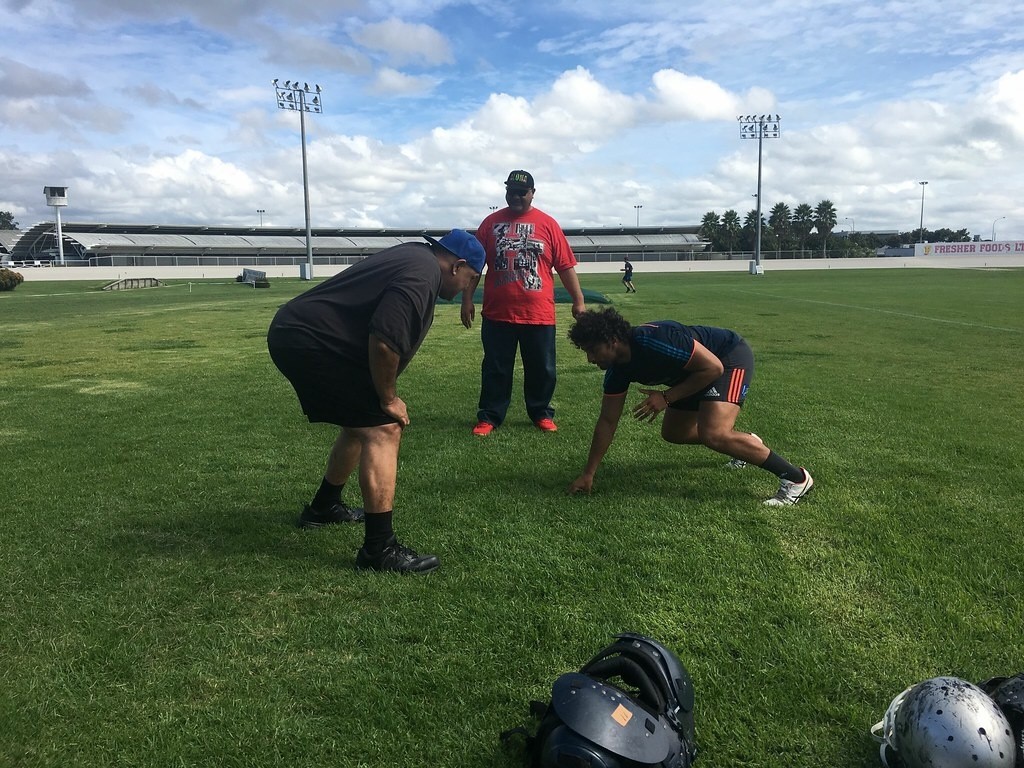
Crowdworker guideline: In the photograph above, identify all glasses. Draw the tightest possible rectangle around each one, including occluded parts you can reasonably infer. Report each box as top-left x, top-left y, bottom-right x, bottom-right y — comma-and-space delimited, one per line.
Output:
508, 188, 531, 196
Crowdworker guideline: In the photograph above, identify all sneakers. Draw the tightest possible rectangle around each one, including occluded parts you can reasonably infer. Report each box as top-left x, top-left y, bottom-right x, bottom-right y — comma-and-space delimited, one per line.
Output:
300, 502, 366, 528
356, 534, 439, 574
723, 433, 763, 470
473, 422, 493, 436
763, 467, 814, 506
533, 418, 557, 432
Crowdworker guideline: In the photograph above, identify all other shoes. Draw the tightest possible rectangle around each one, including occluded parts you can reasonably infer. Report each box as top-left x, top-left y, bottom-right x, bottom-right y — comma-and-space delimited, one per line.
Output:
632, 289, 636, 293
626, 287, 630, 293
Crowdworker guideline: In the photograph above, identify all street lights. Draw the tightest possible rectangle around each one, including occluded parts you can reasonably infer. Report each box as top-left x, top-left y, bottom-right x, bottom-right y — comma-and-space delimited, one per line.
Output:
737, 114, 781, 266
992, 216, 1005, 241
845, 218, 854, 232
257, 210, 265, 226
634, 205, 642, 227
270, 79, 323, 278
919, 181, 928, 242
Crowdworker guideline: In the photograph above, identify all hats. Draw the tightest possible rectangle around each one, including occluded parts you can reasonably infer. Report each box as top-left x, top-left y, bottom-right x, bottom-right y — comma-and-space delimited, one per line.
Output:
504, 170, 534, 190
422, 229, 487, 273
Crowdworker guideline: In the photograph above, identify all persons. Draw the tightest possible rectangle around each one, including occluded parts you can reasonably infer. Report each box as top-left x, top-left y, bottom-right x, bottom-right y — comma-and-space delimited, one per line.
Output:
569, 307, 813, 507
460, 170, 586, 436
267, 228, 485, 574
620, 257, 636, 293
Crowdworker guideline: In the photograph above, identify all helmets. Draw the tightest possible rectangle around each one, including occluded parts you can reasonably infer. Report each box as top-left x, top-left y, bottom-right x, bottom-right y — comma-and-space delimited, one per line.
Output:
883, 677, 1016, 768
977, 672, 1024, 732
500, 632, 696, 768
